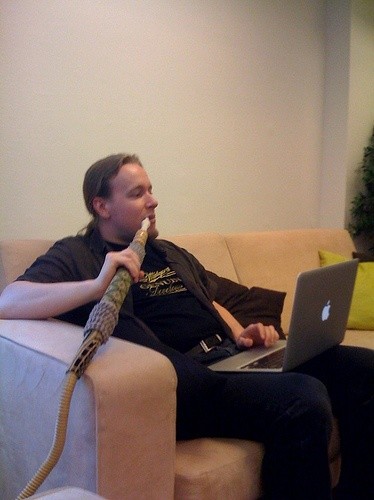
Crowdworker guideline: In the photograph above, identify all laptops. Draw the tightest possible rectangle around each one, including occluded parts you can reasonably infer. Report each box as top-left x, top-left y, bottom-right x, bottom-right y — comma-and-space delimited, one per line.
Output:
207, 259, 359, 373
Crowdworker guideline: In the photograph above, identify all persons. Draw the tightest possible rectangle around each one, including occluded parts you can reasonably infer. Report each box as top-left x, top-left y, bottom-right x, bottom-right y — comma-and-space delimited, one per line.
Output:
0, 152, 374, 500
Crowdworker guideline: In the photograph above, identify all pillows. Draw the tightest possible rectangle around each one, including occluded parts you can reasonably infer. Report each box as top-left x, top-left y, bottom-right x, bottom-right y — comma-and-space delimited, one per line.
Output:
205, 270, 286, 340
317, 248, 374, 331
112, 310, 228, 441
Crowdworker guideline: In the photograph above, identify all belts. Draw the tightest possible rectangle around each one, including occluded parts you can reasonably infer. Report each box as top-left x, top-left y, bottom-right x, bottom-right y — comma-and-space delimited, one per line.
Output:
184, 333, 224, 359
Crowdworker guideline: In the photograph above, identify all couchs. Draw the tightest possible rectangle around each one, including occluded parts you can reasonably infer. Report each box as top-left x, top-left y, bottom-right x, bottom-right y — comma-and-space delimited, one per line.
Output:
1, 230, 373, 500
